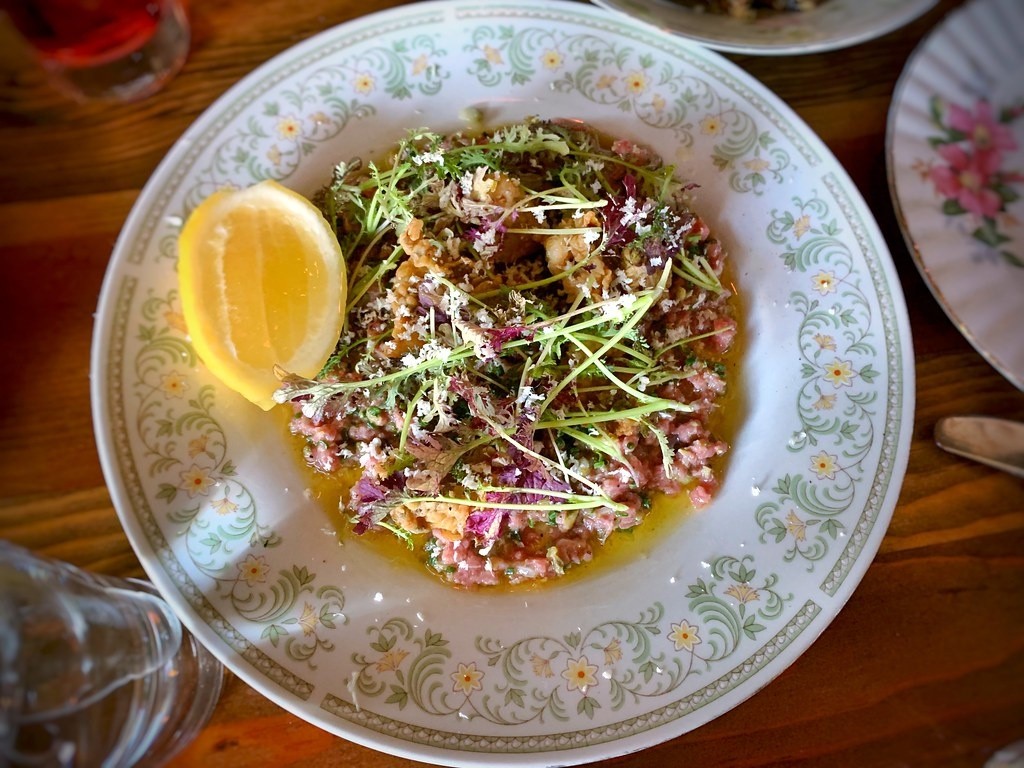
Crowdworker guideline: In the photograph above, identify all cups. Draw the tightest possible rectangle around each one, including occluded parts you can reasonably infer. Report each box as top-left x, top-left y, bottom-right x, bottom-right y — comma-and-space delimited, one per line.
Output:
1, 533, 223, 768
1, 0, 192, 114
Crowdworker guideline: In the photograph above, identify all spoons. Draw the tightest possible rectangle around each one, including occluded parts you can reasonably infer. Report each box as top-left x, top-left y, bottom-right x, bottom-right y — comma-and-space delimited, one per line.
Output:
934, 414, 1024, 477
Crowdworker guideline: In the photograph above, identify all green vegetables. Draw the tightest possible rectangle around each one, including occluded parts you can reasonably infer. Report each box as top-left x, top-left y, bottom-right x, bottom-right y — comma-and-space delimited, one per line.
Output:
274, 131, 733, 549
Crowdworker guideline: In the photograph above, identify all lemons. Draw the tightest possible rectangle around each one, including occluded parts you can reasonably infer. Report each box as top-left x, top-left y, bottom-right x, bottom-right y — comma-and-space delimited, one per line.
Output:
176, 177, 349, 412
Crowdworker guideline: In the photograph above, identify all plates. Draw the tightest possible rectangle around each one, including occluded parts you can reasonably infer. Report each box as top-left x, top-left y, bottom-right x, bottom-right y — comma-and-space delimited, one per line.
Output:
883, 2, 1022, 394
87, 0, 920, 766
593, 1, 944, 59
